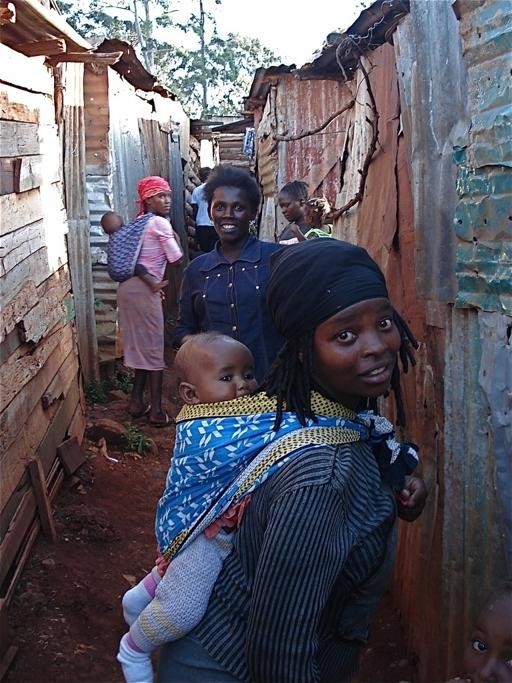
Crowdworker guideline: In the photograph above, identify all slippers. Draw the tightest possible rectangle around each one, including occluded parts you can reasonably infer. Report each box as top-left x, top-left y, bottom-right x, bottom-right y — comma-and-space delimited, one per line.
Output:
133, 403, 174, 428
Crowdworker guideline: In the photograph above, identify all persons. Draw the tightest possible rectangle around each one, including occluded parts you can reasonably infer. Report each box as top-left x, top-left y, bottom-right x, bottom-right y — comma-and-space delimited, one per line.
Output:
99, 212, 169, 293
157, 236, 429, 683
116, 328, 258, 683
290, 198, 334, 243
117, 175, 185, 429
191, 166, 220, 254
444, 584, 512, 683
276, 180, 313, 246
172, 163, 288, 392
